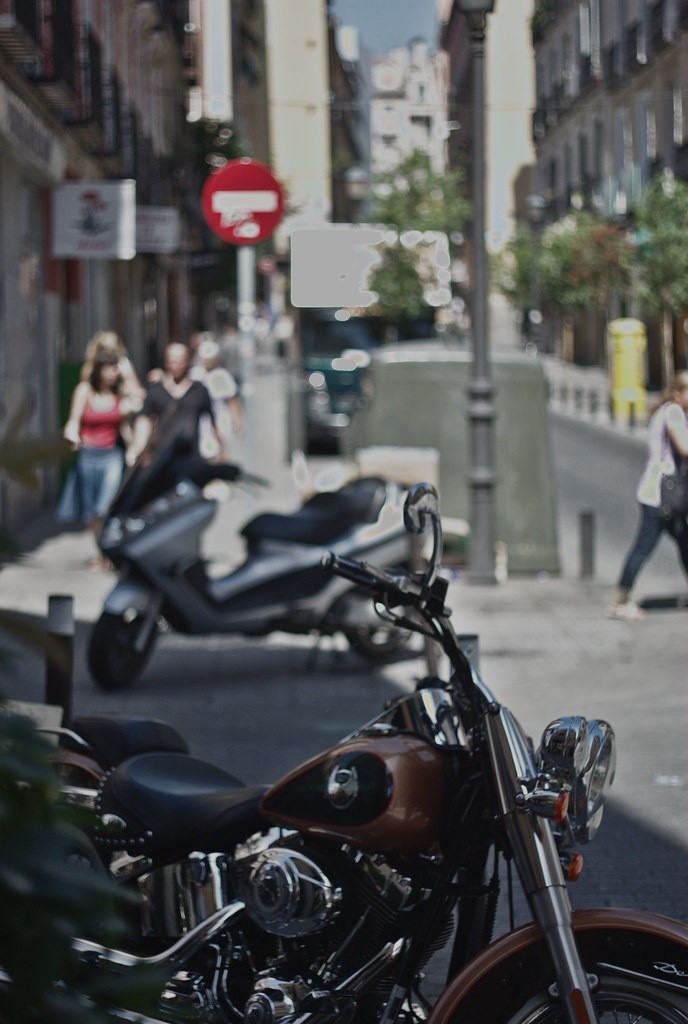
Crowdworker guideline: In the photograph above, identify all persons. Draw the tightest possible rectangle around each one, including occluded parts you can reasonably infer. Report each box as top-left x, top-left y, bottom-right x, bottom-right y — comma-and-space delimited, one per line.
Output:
605, 367, 688, 623
62, 328, 243, 572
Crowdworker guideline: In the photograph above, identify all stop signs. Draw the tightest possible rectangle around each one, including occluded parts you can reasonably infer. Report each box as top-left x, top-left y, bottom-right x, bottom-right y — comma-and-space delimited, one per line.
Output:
204, 154, 287, 245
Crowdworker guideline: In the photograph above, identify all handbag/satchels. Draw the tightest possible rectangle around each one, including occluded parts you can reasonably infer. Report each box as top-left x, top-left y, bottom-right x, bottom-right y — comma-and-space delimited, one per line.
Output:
660, 473, 688, 518
56, 456, 78, 525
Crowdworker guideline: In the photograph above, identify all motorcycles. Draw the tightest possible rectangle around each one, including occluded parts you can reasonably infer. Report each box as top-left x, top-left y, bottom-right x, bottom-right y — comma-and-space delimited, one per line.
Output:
36, 479, 688, 1024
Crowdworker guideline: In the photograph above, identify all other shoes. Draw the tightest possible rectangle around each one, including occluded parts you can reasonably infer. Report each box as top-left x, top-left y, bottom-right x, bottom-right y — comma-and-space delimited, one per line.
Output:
605, 602, 647, 621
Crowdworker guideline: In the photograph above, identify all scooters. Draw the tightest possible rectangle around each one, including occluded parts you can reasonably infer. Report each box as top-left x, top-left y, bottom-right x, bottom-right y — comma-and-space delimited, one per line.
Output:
84, 374, 445, 693
